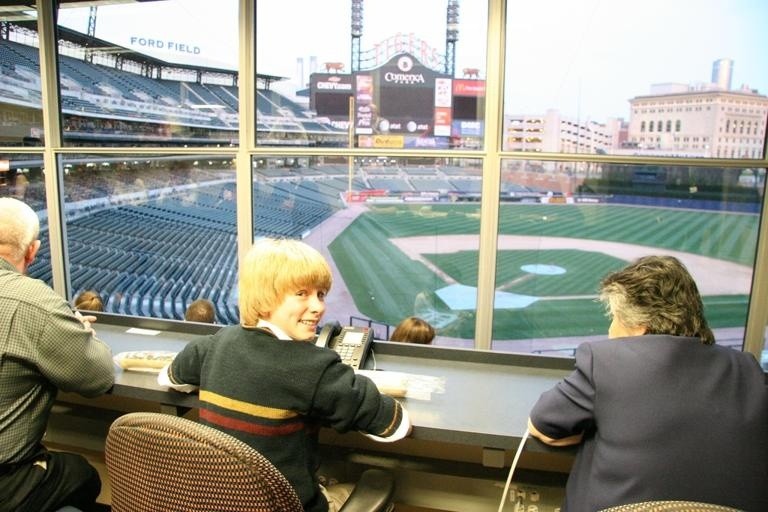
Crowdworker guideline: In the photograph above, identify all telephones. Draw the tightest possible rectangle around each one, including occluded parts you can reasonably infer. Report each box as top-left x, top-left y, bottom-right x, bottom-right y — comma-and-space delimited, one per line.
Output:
315, 319, 374, 371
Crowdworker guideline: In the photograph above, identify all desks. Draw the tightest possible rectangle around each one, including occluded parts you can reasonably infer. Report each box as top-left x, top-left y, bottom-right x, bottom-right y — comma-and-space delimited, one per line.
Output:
36, 316, 593, 511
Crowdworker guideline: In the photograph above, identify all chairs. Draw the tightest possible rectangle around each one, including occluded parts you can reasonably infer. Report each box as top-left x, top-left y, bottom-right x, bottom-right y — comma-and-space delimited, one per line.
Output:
24, 186, 239, 327
104, 411, 396, 512
594, 500, 744, 512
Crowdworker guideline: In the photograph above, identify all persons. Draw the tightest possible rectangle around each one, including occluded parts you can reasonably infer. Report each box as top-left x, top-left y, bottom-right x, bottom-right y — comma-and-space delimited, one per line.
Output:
75, 291, 103, 312
528, 255, 768, 512
0, 196, 117, 512
184, 298, 218, 324
157, 239, 412, 512
391, 316, 435, 345
